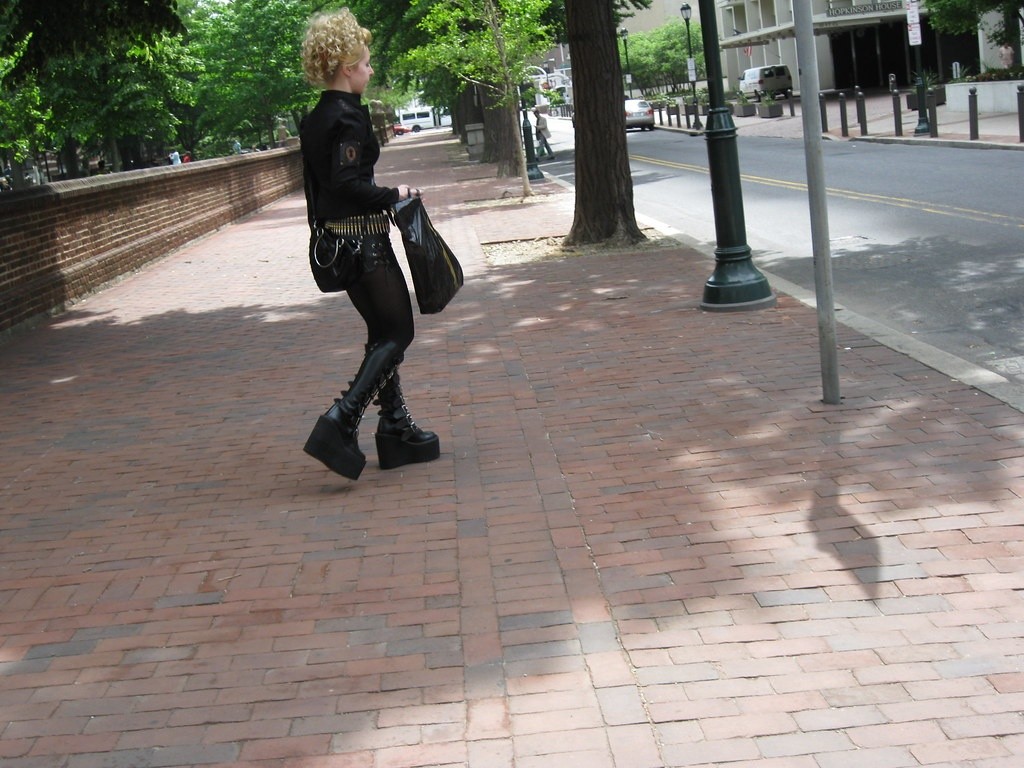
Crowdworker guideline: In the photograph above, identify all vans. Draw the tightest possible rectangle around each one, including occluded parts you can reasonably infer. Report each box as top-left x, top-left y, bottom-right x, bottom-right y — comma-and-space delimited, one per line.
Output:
399, 106, 453, 133
737, 64, 794, 102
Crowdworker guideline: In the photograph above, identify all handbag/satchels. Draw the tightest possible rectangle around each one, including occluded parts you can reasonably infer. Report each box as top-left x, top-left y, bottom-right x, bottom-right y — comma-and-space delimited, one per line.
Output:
394, 188, 464, 314
309, 229, 363, 293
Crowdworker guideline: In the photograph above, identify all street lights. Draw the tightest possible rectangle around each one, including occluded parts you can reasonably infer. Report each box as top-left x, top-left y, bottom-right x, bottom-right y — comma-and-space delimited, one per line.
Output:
680, 1, 704, 130
541, 60, 553, 104
618, 27, 634, 99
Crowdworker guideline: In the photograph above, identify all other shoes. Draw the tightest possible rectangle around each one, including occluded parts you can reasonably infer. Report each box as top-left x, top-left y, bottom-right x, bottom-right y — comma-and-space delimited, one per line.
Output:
547, 155, 554, 160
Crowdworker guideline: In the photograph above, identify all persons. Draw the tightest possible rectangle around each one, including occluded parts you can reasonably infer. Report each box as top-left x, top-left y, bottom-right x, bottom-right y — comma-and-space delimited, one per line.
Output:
999, 42, 1015, 70
534, 109, 555, 161
277, 118, 291, 146
168, 149, 182, 165
233, 139, 242, 154
183, 149, 191, 164
293, 7, 440, 481
98, 156, 106, 172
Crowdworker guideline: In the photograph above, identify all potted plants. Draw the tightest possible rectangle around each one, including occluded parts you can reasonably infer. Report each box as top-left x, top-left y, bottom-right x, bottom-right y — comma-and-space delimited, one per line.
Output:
907, 69, 946, 109
665, 87, 783, 120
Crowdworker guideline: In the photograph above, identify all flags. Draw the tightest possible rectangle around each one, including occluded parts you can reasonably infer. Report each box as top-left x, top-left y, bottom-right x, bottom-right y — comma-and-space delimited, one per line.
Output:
733, 27, 753, 55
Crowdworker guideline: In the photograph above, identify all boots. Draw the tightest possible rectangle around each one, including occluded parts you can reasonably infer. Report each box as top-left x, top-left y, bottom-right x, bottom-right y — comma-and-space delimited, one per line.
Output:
303, 340, 404, 480
365, 342, 440, 469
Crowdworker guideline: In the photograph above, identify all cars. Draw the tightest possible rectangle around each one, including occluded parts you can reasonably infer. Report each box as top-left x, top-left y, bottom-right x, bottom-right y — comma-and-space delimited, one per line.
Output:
624, 99, 655, 131
393, 121, 411, 136
